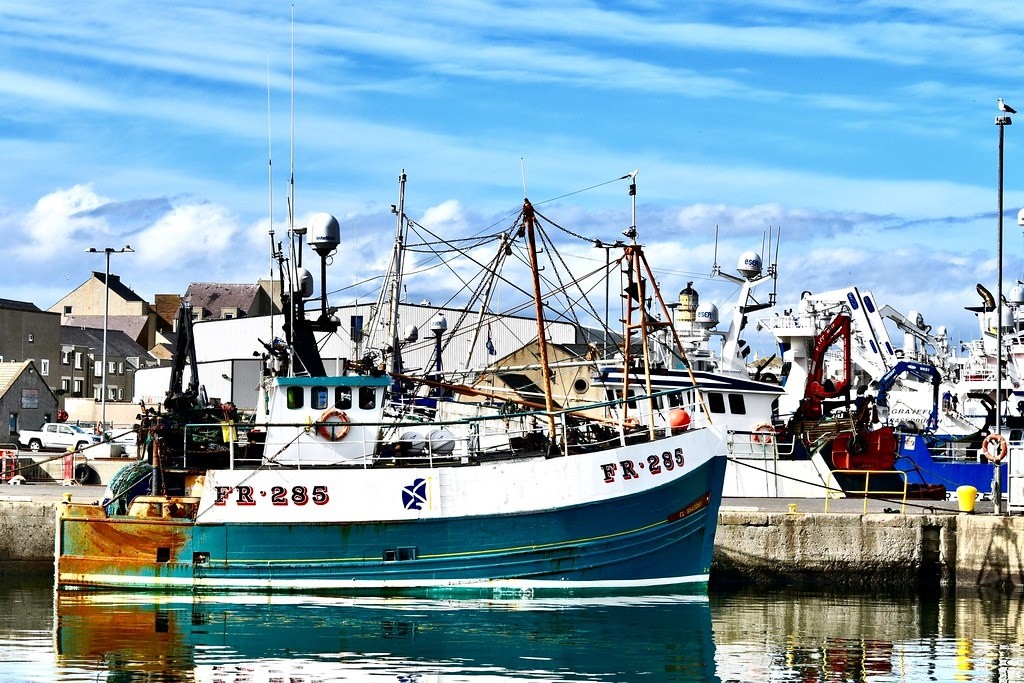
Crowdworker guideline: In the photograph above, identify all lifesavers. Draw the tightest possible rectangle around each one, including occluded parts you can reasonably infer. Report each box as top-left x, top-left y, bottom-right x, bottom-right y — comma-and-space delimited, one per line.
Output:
320, 409, 350, 441
761, 373, 777, 383
894, 349, 905, 359
753, 424, 774, 446
846, 435, 868, 455
982, 433, 1007, 462
74, 463, 93, 484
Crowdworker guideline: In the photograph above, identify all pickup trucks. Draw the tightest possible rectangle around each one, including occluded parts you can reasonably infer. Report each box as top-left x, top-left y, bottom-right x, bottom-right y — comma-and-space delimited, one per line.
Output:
20, 422, 101, 452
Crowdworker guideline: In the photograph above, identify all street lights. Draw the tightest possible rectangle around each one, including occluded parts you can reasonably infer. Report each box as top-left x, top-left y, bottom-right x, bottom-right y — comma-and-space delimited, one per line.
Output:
82, 244, 135, 442
995, 97, 1018, 450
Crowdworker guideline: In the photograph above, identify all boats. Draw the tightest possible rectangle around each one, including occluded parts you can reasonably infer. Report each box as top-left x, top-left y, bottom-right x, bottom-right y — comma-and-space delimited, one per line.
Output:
16, 1, 1018, 604
